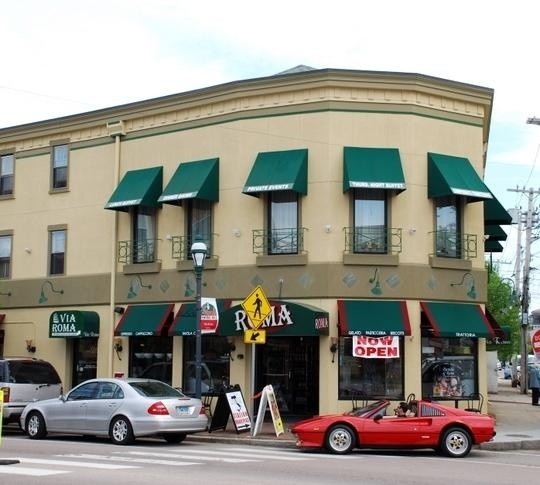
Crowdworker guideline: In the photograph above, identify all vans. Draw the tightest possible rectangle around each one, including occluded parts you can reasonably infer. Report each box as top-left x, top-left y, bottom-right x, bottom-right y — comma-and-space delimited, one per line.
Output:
419, 355, 475, 398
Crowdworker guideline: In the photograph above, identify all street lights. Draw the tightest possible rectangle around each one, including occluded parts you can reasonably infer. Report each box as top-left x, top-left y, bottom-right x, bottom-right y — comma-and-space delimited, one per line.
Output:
190, 242, 208, 396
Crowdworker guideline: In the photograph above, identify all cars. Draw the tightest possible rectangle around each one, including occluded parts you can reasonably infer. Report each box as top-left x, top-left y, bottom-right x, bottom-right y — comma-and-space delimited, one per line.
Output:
135, 360, 214, 395
19, 378, 208, 444
504, 355, 540, 387
0, 356, 64, 429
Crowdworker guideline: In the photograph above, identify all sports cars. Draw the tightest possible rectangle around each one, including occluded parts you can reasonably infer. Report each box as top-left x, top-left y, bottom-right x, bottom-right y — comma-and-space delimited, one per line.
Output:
290, 398, 496, 457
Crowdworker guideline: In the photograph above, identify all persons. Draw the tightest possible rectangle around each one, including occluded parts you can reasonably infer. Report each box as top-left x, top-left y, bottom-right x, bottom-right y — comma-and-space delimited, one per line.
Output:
398, 402, 408, 417
529, 364, 540, 406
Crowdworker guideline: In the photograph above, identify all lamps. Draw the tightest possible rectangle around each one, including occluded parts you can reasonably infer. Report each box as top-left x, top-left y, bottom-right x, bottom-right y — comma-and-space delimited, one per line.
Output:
26, 338, 33, 352
450, 273, 480, 300
126, 275, 151, 300
330, 336, 339, 363
369, 268, 383, 296
38, 280, 63, 305
114, 337, 123, 354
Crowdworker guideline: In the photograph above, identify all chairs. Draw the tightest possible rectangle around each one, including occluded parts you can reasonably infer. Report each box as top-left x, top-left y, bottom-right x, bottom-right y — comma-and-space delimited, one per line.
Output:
351, 389, 485, 418
200, 389, 215, 420
